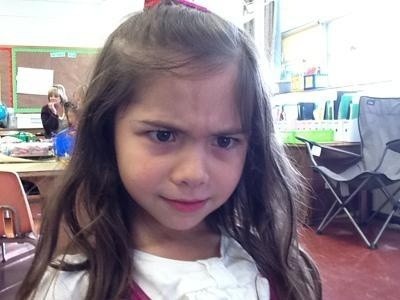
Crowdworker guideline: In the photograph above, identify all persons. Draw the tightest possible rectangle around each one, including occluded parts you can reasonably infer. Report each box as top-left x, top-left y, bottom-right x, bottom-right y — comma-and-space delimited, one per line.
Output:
14, 0, 322, 299
40, 83, 69, 140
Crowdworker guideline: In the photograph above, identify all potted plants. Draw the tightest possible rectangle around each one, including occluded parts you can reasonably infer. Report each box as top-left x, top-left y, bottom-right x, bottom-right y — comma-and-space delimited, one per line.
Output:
277, 58, 291, 94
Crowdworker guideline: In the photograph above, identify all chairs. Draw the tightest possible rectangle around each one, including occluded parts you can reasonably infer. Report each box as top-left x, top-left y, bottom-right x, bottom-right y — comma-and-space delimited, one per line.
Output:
294, 97, 400, 249
0, 169, 48, 262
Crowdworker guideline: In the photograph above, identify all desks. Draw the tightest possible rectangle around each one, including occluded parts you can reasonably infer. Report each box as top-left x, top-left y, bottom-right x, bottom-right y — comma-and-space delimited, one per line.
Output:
281, 141, 372, 227
0, 129, 71, 204
